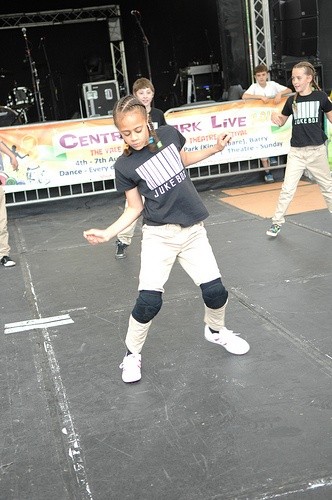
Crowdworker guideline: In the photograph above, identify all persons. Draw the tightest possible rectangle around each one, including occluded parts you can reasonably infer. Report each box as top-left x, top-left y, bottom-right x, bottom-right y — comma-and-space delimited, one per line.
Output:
242, 66, 292, 183
266, 61, 332, 237
115, 77, 167, 259
83, 94, 250, 383
0, 139, 18, 267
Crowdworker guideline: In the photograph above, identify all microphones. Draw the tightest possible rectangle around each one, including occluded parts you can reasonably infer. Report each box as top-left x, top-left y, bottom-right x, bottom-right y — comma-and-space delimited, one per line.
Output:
131, 10, 139, 15
22, 28, 26, 37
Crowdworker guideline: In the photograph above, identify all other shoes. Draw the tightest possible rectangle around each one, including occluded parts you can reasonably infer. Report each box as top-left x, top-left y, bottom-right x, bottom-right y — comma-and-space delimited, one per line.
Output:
271, 158, 278, 164
265, 175, 273, 182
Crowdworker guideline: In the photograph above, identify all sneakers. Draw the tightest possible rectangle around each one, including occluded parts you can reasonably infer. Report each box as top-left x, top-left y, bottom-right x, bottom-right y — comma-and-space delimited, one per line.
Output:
115, 239, 130, 258
204, 324, 250, 355
1, 255, 16, 266
119, 351, 141, 382
266, 224, 280, 236
